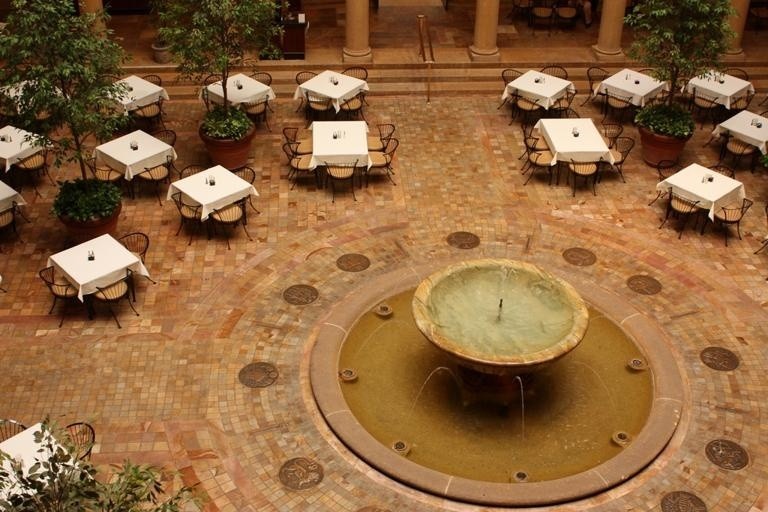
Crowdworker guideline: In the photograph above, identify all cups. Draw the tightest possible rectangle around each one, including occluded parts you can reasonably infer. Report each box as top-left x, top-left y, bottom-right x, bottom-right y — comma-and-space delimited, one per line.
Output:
0, 78, 339, 260
533, 77, 764, 183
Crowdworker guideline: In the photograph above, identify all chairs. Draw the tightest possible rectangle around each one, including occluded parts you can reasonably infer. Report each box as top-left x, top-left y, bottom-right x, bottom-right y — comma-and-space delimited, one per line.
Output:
506, 0, 596, 38
0, 418, 95, 511
